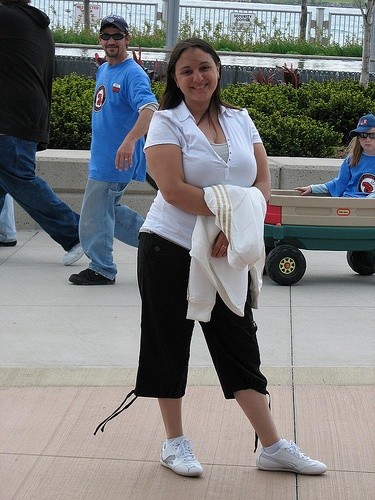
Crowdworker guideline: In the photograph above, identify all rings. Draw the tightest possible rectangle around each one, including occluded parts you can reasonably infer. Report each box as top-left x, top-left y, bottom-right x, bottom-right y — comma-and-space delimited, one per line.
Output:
125, 159, 128, 160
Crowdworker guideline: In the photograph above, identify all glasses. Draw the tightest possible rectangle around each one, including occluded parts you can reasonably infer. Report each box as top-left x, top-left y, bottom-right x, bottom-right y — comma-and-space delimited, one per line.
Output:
99, 32, 127, 41
359, 132, 375, 139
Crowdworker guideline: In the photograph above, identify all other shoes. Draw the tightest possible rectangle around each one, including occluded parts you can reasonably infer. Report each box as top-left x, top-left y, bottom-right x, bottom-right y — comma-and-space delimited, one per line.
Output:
0, 240, 17, 246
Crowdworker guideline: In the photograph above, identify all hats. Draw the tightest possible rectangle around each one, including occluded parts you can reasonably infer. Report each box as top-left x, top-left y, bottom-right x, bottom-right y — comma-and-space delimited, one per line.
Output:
348, 113, 375, 137
99, 14, 129, 32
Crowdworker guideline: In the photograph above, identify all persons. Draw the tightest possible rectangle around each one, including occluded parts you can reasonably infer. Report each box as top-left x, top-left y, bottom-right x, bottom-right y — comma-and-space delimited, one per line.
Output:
0, 0, 85, 266
294, 113, 375, 198
133, 38, 327, 477
223, 245, 227, 249
68, 14, 160, 285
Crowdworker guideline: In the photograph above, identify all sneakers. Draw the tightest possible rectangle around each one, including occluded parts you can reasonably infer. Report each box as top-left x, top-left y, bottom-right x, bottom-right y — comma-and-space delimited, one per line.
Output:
69, 268, 116, 285
159, 437, 203, 478
62, 242, 85, 265
255, 439, 326, 475
